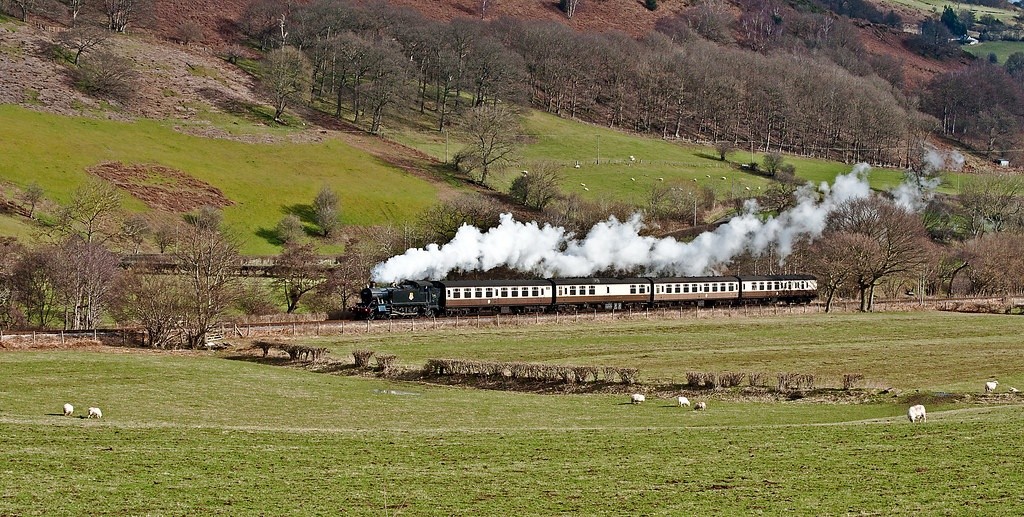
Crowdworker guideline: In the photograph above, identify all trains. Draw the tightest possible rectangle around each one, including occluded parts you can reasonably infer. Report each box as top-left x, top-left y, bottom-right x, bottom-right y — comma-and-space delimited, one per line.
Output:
350, 274, 818, 320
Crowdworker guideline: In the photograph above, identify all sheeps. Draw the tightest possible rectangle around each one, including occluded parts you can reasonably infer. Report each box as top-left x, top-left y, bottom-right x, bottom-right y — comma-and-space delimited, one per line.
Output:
629, 393, 646, 405
677, 396, 691, 408
907, 404, 928, 424
63, 403, 103, 420
694, 402, 708, 412
985, 380, 1018, 394
